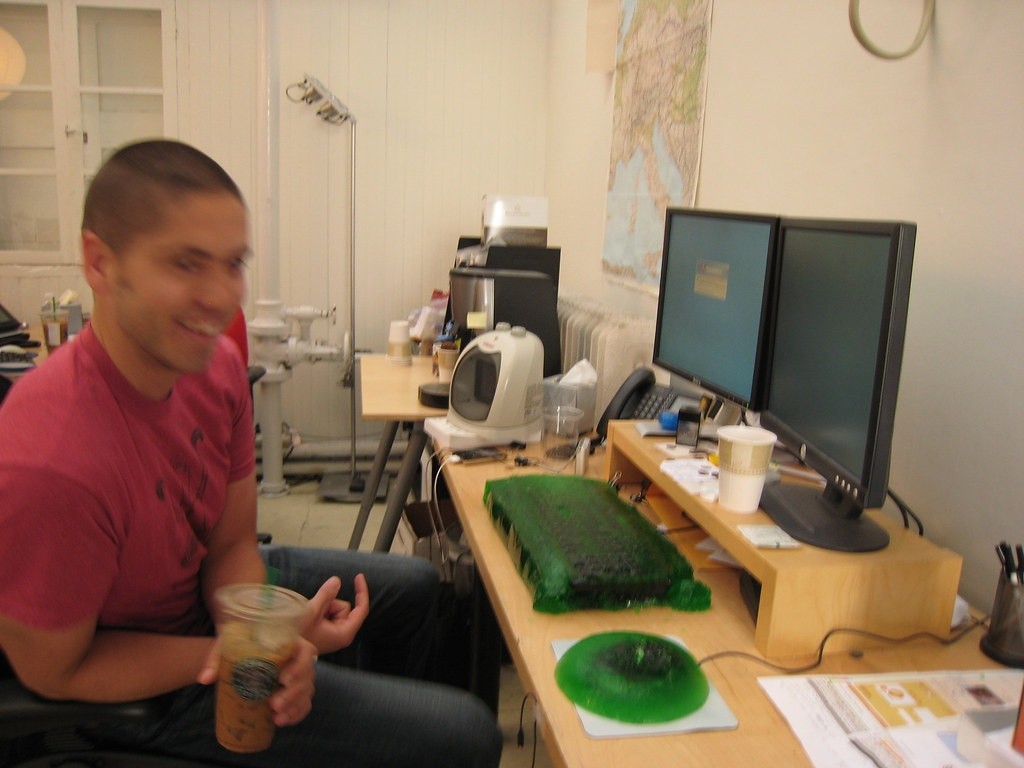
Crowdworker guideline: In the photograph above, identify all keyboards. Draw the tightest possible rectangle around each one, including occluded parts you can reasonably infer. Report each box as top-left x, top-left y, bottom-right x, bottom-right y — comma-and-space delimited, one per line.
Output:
519, 479, 673, 596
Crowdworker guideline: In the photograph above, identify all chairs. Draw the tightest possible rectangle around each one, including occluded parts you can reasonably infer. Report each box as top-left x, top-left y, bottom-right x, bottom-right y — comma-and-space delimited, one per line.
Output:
0, 370, 202, 768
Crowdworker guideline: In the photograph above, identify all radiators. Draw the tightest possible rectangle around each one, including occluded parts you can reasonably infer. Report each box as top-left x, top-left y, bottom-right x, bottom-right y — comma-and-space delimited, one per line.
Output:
558, 299, 624, 430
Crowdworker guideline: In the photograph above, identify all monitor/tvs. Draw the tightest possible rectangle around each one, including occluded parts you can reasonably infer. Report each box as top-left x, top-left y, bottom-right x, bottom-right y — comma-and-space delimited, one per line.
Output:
652, 207, 917, 554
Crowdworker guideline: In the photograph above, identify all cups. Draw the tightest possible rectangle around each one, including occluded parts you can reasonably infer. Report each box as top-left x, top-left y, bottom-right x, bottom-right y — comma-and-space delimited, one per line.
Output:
540, 405, 583, 464
40, 310, 70, 357
213, 586, 308, 753
437, 348, 457, 382
987, 568, 1024, 656
385, 321, 413, 367
717, 425, 776, 514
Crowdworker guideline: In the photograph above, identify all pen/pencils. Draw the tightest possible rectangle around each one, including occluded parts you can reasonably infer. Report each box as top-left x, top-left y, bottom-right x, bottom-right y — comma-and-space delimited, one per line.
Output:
993, 539, 1024, 583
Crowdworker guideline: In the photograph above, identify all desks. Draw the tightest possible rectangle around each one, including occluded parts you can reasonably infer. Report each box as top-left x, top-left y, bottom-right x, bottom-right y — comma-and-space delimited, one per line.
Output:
427, 418, 1024, 768
600, 417, 962, 657
345, 353, 456, 553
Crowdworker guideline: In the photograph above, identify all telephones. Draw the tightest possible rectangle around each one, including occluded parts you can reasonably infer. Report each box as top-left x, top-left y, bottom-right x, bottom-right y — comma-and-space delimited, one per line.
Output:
597, 367, 712, 443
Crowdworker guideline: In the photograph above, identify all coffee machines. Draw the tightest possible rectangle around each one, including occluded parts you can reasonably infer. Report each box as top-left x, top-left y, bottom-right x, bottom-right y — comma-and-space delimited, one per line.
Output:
418, 267, 562, 409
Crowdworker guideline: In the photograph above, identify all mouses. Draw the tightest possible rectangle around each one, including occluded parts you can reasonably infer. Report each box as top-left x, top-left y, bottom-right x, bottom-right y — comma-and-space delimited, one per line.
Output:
606, 637, 676, 679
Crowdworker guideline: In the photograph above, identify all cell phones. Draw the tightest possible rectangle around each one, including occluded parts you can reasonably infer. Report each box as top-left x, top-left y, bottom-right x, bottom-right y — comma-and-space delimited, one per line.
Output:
451, 446, 507, 465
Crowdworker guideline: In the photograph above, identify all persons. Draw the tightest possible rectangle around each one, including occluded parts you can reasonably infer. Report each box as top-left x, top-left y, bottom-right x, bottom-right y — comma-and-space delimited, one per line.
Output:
572, 248, 574, 253
1, 138, 505, 768
